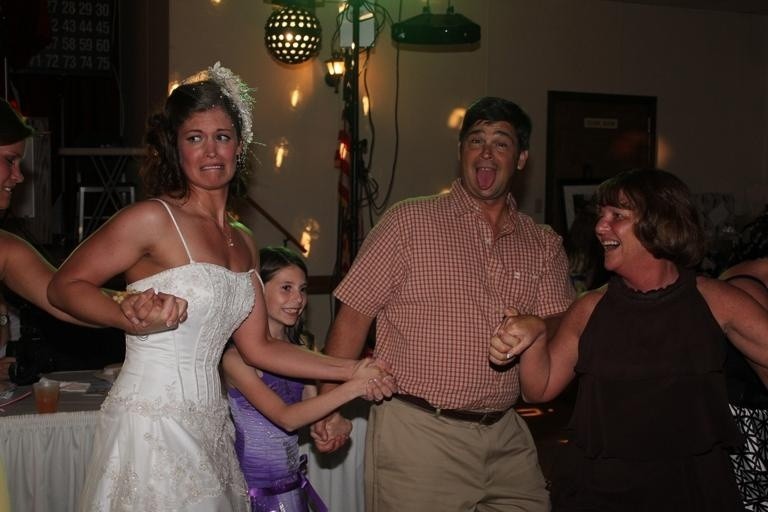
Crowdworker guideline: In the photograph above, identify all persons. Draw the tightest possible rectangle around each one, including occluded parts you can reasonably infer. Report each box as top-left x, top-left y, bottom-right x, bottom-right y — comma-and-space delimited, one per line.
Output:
314, 97, 576, 511
0, 95, 188, 332
218, 246, 394, 512
0, 355, 18, 384
41, 59, 397, 512
487, 163, 766, 511
557, 233, 593, 295
0, 291, 11, 349
712, 204, 767, 511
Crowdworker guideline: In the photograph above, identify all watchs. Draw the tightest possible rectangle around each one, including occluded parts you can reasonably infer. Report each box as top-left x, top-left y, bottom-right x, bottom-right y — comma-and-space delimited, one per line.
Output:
0, 312, 10, 329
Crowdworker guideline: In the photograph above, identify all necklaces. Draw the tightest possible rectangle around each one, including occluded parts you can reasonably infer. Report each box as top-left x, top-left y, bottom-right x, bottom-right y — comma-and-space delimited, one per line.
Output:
192, 196, 234, 246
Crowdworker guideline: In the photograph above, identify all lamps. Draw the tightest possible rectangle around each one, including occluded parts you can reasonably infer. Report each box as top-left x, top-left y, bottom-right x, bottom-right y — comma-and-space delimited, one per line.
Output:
263, 0, 326, 65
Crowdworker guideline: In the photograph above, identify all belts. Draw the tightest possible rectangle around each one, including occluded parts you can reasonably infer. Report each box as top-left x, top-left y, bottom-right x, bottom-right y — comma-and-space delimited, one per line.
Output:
392, 393, 504, 425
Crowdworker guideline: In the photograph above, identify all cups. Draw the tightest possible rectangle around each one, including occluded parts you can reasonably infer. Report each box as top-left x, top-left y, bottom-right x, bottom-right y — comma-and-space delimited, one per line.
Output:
33, 381, 60, 413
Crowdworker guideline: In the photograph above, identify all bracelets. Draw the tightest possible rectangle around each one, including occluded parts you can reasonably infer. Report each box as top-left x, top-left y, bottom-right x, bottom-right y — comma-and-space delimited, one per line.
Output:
112, 289, 134, 307
131, 321, 150, 342
